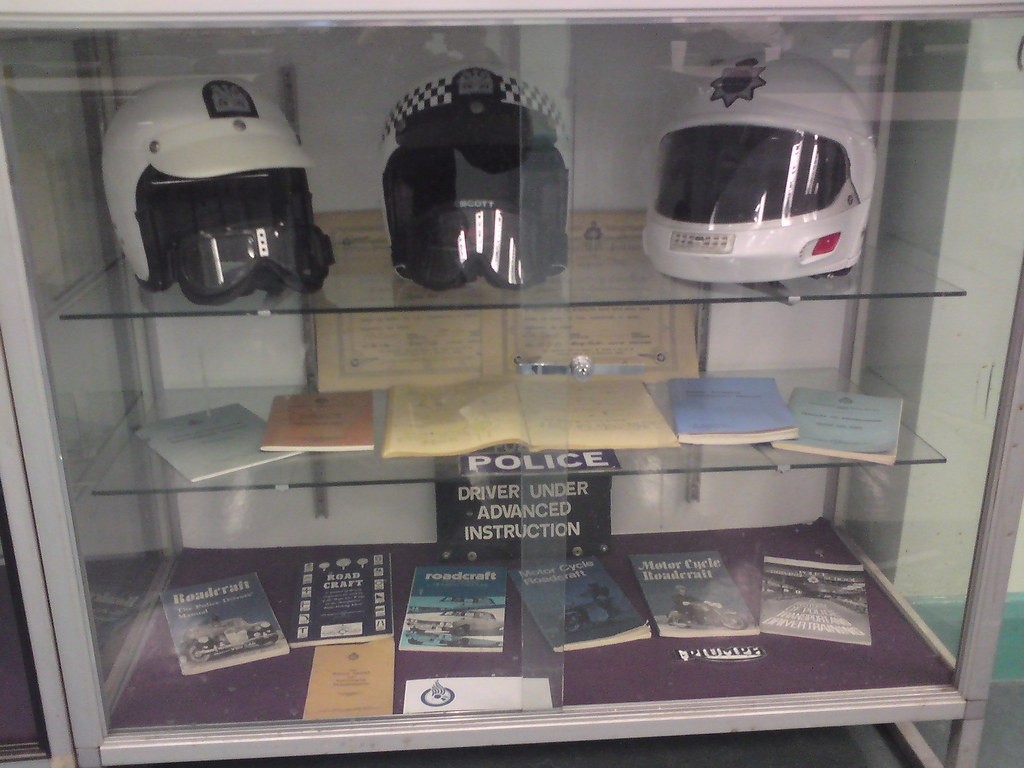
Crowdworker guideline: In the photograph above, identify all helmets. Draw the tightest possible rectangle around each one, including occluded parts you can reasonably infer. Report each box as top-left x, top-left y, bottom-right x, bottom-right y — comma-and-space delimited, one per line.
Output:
100, 75, 334, 302
375, 63, 569, 292
643, 49, 879, 284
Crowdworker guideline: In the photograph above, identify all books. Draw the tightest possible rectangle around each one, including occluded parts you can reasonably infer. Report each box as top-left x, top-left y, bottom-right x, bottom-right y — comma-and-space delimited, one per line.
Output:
398, 564, 507, 652
260, 390, 375, 451
159, 572, 290, 676
507, 555, 652, 652
759, 556, 872, 647
772, 387, 903, 465
665, 377, 799, 447
629, 551, 760, 637
287, 552, 392, 648
381, 375, 681, 456
131, 403, 304, 483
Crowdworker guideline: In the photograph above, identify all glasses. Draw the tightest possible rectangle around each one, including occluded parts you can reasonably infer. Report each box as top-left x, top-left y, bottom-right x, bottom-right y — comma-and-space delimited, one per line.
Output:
397, 196, 555, 290
173, 220, 331, 302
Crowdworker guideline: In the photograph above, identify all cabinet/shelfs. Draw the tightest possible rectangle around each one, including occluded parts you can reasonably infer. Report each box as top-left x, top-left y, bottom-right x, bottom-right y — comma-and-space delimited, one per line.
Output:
0, 1, 1024, 767
